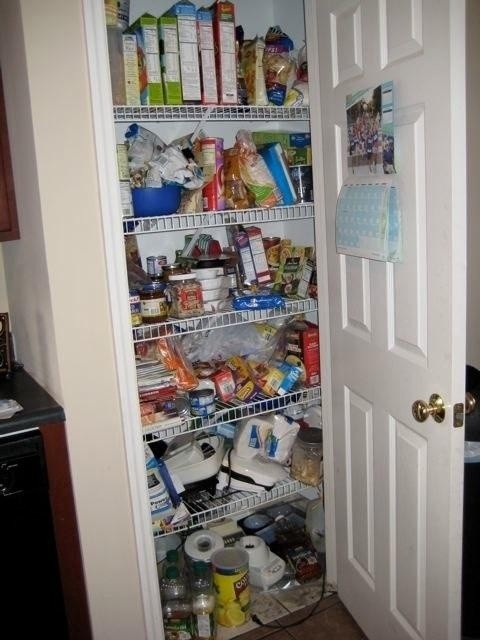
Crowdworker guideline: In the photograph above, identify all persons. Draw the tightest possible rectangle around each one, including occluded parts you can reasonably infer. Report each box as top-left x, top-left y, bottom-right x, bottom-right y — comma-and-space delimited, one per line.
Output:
349, 109, 395, 175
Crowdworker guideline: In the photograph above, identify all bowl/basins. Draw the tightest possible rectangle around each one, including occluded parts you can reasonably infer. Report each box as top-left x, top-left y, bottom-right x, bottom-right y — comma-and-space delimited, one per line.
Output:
130, 184, 182, 218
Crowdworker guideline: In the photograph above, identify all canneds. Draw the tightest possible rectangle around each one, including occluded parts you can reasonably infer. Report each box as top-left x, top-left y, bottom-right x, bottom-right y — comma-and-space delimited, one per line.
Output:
262, 236, 281, 253
286, 533, 323, 585
116, 143, 134, 219
288, 163, 313, 203
224, 147, 242, 181
289, 426, 323, 487
127, 256, 204, 327
155, 388, 216, 417
210, 547, 252, 628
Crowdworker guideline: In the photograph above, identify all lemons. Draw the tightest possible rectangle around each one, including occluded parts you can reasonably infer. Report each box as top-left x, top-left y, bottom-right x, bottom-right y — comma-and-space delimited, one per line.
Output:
214, 601, 246, 629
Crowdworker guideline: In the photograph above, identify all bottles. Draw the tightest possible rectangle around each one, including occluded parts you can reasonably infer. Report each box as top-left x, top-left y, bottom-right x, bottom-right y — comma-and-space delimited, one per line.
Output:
159, 549, 216, 639
165, 273, 206, 319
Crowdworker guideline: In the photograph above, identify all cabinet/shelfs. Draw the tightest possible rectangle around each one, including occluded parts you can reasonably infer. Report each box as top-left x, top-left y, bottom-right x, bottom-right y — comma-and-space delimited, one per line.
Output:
102, 0, 333, 638
